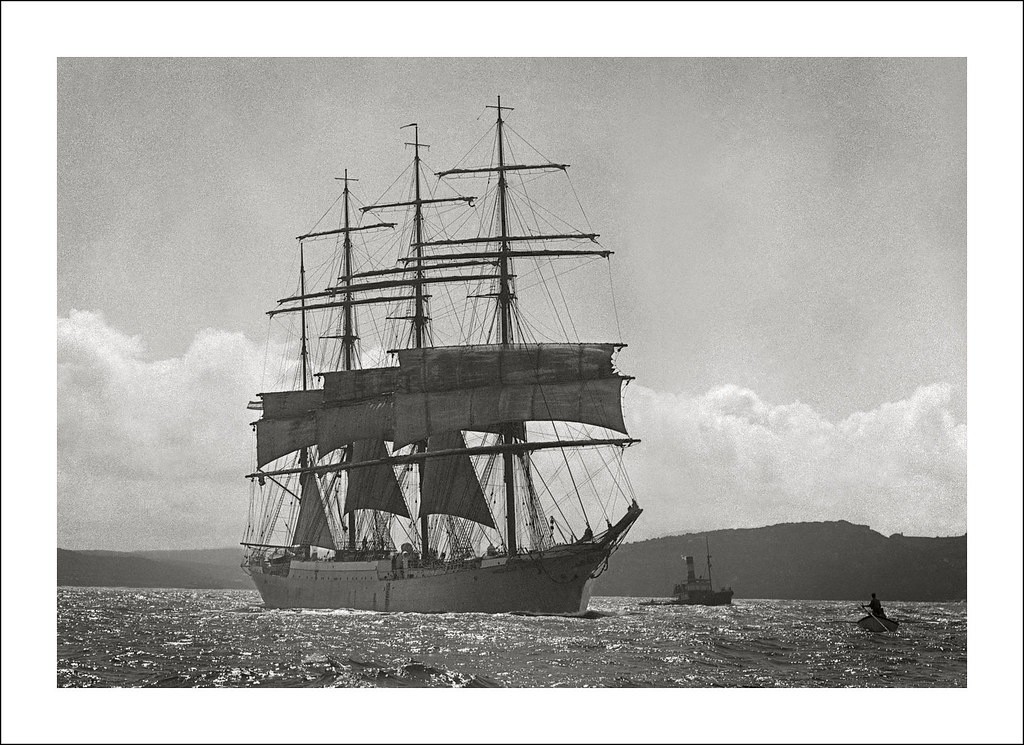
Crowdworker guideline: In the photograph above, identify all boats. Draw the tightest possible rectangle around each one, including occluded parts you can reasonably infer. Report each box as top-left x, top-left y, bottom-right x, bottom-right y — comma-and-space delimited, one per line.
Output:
857, 615, 900, 633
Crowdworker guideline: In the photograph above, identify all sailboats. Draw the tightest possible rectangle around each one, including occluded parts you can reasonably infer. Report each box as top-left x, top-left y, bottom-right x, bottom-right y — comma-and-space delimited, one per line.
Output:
639, 536, 734, 606
240, 95, 643, 613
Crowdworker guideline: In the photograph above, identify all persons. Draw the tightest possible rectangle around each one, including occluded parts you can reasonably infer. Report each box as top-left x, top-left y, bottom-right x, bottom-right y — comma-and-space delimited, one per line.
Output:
863, 593, 881, 616
